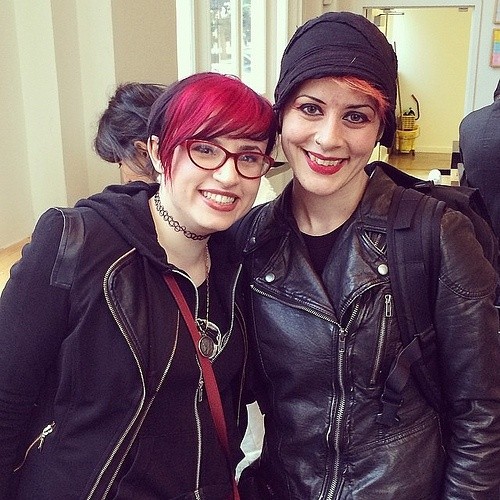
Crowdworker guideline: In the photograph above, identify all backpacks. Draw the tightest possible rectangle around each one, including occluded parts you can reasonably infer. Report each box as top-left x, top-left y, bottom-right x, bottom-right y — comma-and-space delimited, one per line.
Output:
364, 160, 500, 429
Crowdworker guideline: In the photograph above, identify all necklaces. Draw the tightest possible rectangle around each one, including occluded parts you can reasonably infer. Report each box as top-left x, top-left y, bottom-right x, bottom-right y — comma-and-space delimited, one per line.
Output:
142, 191, 216, 359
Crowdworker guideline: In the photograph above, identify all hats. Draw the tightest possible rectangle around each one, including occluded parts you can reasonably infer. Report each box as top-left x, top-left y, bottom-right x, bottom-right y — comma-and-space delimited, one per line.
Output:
273, 11, 399, 148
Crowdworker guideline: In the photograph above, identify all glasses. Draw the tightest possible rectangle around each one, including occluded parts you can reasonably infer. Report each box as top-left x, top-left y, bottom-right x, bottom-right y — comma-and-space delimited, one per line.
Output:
182, 139, 274, 180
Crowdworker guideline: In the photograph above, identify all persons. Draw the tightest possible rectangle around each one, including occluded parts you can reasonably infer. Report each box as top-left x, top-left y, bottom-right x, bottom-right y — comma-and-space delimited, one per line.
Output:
0, 73, 278, 500
459, 78, 500, 236
95, 82, 277, 484
231, 11, 500, 500
